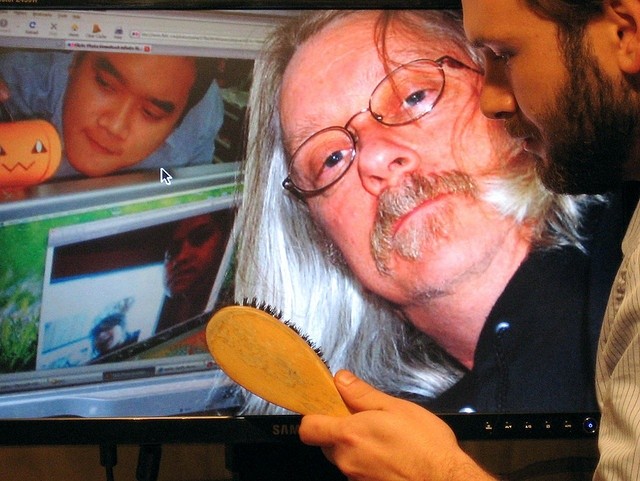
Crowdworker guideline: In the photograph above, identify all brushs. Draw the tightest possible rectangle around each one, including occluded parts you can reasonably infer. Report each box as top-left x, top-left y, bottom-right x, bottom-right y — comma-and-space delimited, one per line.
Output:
204, 297, 352, 416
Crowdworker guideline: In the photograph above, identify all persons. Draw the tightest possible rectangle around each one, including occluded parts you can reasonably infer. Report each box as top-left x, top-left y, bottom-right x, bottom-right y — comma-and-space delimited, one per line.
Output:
2, 50, 228, 178
205, 8, 620, 416
162, 209, 228, 322
90, 315, 143, 351
299, 0, 640, 476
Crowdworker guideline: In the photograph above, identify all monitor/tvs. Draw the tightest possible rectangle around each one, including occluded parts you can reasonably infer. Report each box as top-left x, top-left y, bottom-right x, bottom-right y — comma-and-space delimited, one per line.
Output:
1, 0, 640, 450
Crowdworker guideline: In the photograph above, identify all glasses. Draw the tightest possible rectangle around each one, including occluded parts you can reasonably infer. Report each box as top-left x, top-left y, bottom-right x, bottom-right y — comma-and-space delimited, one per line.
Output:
281, 54, 484, 200
